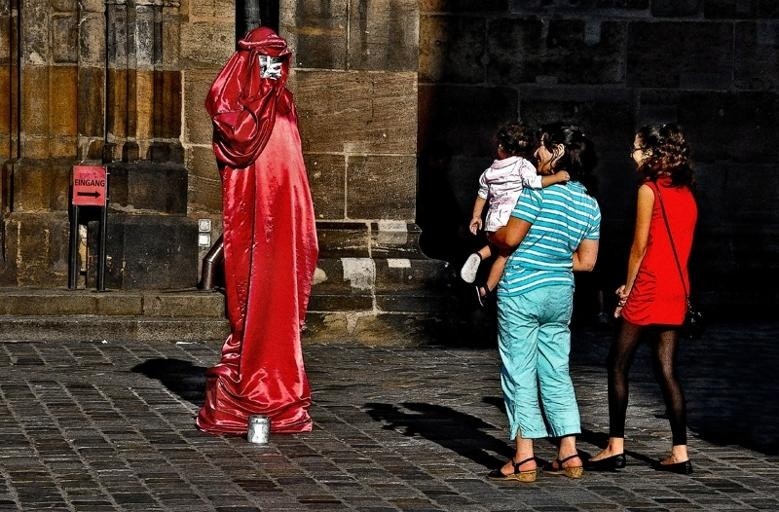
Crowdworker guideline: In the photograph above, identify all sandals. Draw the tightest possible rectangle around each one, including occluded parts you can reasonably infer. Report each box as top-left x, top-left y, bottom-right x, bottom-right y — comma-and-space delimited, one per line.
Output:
476, 285, 490, 307
460, 253, 481, 283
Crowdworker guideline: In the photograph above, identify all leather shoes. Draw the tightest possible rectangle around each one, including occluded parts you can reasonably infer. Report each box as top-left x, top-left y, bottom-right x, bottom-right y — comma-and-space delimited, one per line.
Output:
585, 454, 626, 470
652, 460, 692, 475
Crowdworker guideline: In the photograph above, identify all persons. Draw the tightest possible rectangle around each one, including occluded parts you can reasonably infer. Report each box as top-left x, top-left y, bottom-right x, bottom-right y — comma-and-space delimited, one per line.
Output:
485, 117, 602, 484
460, 120, 570, 310
582, 120, 699, 473
197, 24, 322, 437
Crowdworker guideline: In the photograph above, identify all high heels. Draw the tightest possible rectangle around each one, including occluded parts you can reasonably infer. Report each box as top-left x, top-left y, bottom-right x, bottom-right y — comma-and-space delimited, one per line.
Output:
543, 454, 583, 479
487, 457, 537, 482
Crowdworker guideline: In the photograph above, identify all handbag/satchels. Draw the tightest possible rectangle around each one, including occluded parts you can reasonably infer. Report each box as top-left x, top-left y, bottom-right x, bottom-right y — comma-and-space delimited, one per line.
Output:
684, 309, 702, 339
